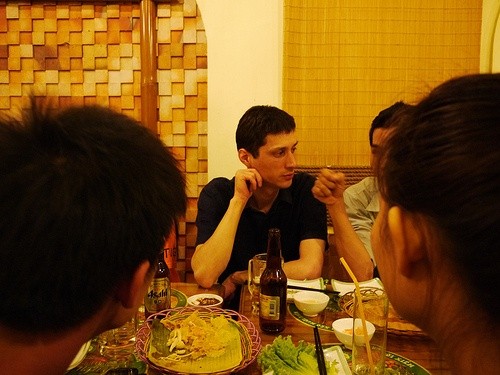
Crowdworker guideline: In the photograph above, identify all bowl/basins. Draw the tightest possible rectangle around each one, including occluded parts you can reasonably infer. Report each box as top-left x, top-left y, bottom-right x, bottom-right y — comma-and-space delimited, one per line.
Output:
294, 291, 329, 317
331, 318, 375, 350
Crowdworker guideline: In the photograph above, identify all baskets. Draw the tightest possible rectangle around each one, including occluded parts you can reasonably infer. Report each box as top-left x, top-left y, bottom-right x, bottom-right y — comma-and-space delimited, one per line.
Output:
134, 306, 262, 375
338, 286, 429, 338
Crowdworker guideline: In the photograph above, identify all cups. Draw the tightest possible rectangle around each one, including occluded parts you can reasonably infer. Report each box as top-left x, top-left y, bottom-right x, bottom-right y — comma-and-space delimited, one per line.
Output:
248, 254, 285, 317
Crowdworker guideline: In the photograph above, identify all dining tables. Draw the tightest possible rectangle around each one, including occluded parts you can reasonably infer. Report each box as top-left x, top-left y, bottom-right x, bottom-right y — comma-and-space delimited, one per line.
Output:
65, 281, 449, 375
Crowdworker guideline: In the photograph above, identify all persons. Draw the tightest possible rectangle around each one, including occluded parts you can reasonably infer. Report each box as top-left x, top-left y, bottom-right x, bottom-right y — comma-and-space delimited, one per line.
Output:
368, 73, 500, 375
313, 101, 416, 284
192, 104, 326, 289
0, 92, 192, 375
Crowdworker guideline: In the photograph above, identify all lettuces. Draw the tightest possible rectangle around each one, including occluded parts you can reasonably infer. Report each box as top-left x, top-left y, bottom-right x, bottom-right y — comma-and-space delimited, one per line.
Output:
257, 335, 340, 375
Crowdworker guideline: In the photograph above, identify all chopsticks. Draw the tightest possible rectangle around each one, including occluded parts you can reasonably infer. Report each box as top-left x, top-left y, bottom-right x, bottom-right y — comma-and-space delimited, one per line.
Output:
287, 285, 340, 295
313, 326, 328, 375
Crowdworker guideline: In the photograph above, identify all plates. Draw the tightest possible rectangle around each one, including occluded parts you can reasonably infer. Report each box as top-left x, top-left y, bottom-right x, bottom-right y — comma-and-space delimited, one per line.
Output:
286, 278, 325, 303
187, 294, 224, 308
170, 287, 187, 308
323, 346, 352, 375
331, 277, 387, 301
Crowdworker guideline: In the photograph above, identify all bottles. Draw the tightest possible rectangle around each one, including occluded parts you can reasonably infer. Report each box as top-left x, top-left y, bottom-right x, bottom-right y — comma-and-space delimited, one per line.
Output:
143, 250, 172, 324
350, 286, 389, 375
260, 229, 287, 334
